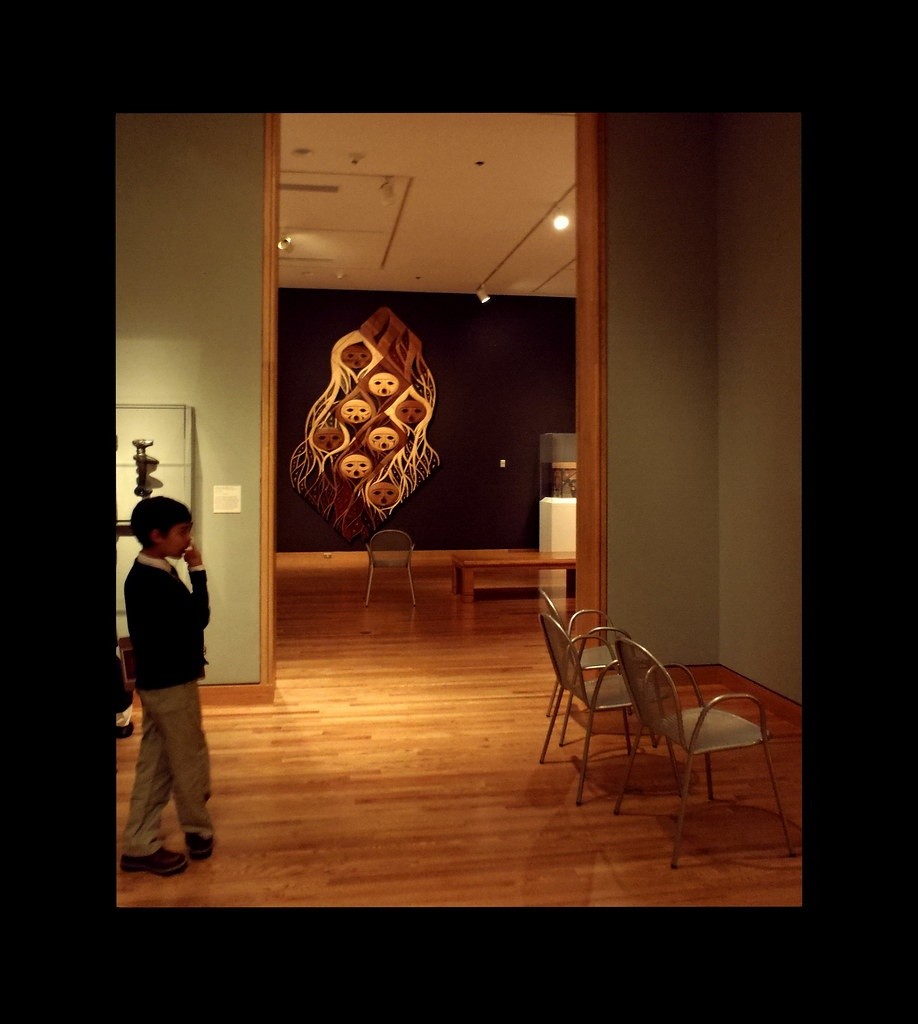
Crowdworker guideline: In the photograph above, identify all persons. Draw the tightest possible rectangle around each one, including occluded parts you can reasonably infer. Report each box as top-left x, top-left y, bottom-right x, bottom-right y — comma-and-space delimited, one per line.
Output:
120, 497, 212, 874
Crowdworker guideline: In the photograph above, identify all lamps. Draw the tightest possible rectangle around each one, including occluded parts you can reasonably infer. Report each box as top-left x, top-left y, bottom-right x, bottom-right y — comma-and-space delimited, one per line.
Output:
476, 288, 490, 304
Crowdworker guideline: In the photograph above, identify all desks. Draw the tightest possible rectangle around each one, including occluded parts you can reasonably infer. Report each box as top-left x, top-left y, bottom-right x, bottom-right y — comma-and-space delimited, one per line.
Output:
451, 551, 576, 603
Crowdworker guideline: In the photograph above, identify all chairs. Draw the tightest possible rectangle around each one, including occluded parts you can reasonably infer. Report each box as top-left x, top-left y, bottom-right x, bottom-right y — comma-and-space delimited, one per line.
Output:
363, 530, 416, 608
538, 584, 797, 870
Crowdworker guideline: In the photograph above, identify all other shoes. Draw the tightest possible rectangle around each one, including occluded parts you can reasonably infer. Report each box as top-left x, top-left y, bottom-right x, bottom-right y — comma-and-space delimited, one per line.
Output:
120, 844, 190, 876
184, 831, 214, 860
